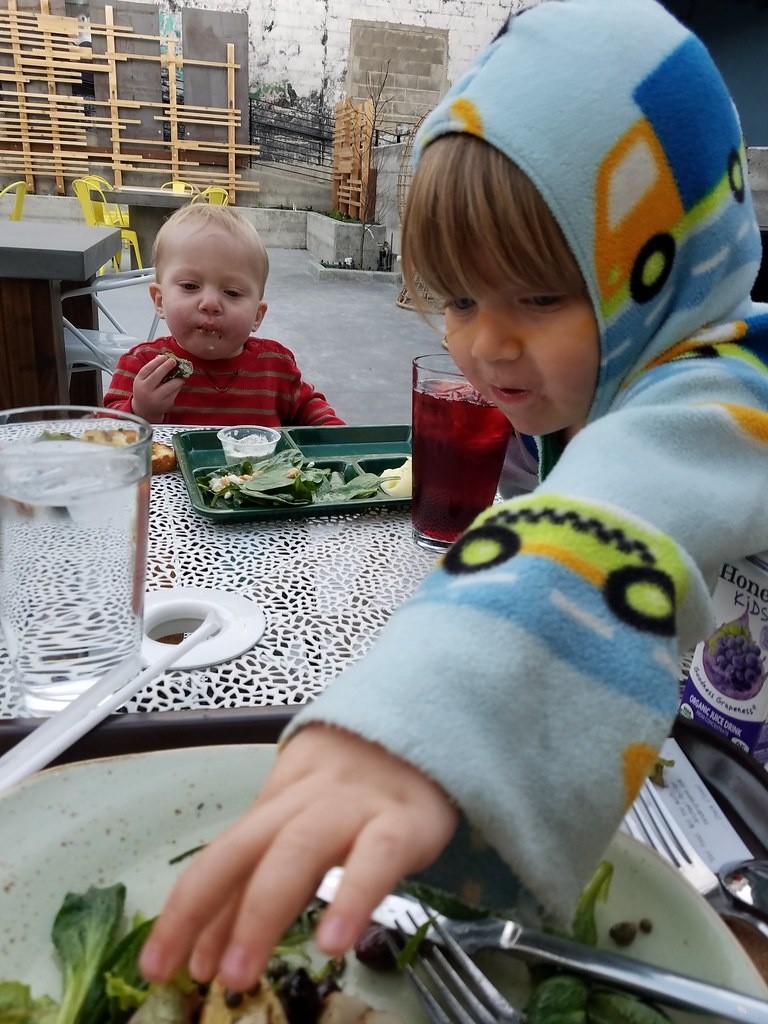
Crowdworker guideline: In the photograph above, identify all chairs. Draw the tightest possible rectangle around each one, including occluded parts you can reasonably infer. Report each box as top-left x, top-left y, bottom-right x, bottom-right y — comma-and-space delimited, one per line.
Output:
40, 267, 161, 418
71, 174, 145, 278
160, 181, 230, 207
0, 181, 27, 220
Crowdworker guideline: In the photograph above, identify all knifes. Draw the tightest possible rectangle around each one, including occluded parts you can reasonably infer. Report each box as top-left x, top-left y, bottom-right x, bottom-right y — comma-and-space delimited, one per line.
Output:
314, 864, 768, 1024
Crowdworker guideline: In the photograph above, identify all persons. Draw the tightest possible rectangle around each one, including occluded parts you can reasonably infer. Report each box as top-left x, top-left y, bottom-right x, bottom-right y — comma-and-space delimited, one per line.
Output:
93, 204, 347, 428
137, 0, 768, 993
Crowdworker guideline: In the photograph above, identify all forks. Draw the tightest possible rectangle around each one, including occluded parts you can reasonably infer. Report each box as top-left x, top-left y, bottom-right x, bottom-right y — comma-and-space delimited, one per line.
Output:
383, 900, 528, 1024
623, 775, 768, 937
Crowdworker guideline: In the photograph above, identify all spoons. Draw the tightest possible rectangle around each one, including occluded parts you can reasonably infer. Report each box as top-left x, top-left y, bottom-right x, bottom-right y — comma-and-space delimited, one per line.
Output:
717, 858, 767, 913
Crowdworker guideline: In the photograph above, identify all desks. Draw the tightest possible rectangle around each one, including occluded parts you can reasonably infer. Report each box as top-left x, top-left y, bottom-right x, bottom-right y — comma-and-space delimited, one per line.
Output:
89, 189, 203, 279
0, 219, 123, 426
0, 423, 766, 911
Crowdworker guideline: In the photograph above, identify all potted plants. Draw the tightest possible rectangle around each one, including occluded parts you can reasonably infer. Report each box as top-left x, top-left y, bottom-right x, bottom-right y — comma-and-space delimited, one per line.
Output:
306, 208, 388, 269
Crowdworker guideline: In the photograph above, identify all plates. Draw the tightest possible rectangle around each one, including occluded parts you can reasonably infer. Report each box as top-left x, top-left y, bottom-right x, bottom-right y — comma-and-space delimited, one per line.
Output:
0, 742, 768, 1024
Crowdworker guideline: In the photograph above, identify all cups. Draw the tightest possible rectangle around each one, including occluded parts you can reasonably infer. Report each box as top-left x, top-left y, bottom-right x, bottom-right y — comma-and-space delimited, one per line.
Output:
411, 354, 513, 554
0, 404, 153, 727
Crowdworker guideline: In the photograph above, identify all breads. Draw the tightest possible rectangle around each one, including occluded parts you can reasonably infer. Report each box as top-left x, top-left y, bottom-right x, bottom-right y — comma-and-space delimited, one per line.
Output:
80, 428, 176, 475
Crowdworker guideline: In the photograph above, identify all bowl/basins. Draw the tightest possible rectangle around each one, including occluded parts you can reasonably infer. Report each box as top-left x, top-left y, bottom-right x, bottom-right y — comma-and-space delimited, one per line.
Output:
217, 425, 281, 465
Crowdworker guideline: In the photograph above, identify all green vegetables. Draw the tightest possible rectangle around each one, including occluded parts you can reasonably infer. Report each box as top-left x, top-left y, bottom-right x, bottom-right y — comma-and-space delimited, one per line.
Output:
196, 449, 403, 509
0, 835, 675, 1024
